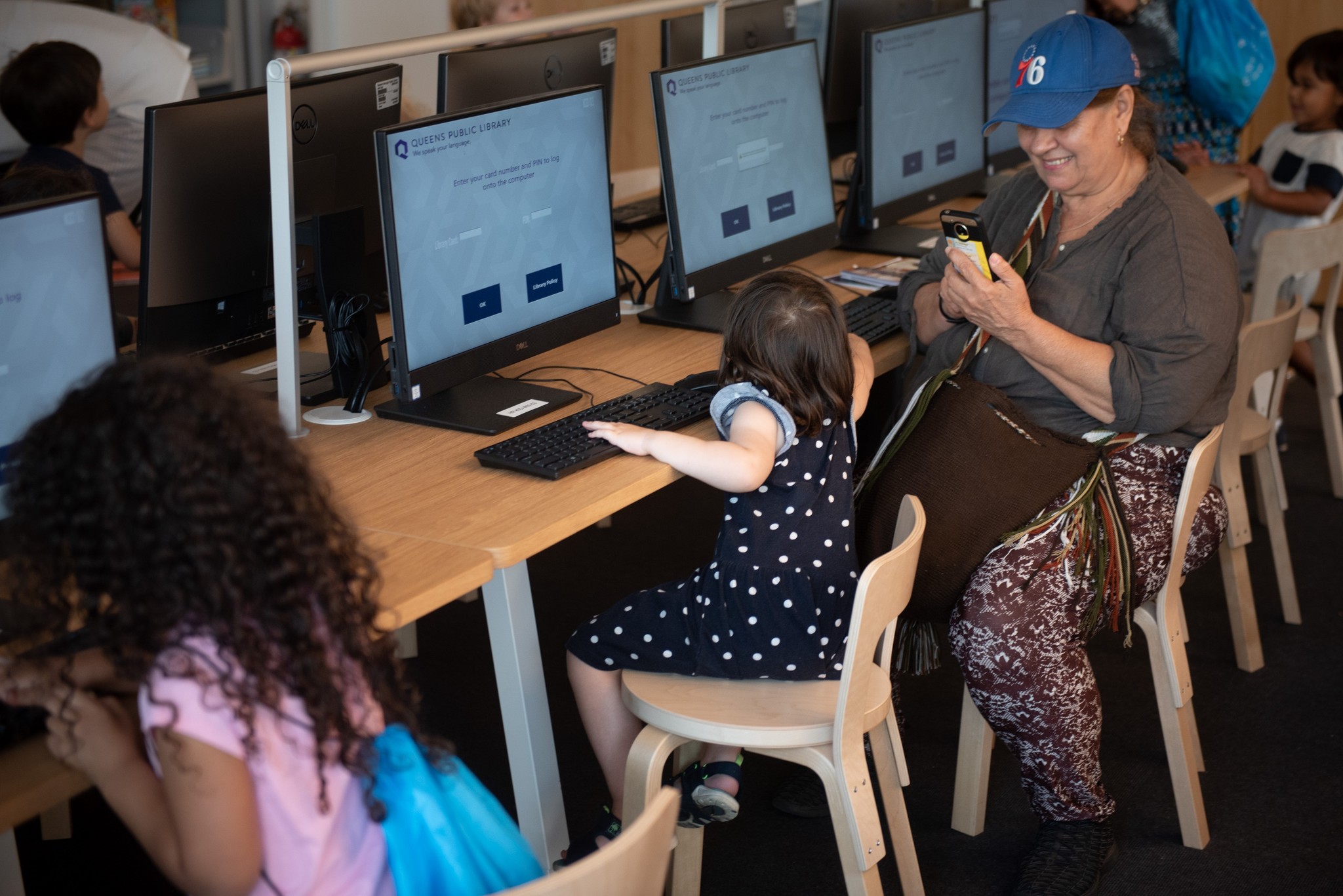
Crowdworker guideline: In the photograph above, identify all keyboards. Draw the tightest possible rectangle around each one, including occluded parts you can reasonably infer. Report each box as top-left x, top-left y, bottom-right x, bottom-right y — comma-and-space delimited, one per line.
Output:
175, 319, 315, 364
837, 295, 902, 347
474, 382, 715, 481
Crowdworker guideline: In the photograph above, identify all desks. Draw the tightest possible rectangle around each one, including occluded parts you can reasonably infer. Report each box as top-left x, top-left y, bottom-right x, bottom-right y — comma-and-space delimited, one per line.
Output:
1, 131, 1242, 895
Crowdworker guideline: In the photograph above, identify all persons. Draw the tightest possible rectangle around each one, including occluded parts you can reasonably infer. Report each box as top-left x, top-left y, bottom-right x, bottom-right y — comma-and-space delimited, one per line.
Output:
553, 267, 874, 870
0, 343, 399, 895
1170, 29, 1343, 449
0, 0, 202, 349
878, 9, 1237, 895
450, 0, 533, 48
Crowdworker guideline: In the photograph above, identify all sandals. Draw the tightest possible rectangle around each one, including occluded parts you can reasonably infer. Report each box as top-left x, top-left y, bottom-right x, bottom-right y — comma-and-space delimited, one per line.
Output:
677, 761, 742, 829
552, 802, 678, 872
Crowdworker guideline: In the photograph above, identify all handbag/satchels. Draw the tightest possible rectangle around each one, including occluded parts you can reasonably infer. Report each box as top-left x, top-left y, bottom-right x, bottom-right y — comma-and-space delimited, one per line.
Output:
860, 373, 1095, 612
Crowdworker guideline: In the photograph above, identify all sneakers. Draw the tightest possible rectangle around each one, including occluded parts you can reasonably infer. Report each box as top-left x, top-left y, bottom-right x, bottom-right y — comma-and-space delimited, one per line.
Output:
1012, 813, 1120, 896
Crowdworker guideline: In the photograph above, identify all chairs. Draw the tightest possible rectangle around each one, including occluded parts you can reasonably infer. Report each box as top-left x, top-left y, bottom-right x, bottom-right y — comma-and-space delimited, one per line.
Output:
436, 220, 1343, 895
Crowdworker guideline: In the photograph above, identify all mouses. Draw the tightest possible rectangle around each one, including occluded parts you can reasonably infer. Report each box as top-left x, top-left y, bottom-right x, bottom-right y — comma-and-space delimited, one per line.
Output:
1167, 158, 1207, 174
674, 369, 724, 395
869, 285, 898, 300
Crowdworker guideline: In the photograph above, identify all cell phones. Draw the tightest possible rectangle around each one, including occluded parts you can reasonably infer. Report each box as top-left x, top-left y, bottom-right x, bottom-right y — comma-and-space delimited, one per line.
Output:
940, 209, 1000, 283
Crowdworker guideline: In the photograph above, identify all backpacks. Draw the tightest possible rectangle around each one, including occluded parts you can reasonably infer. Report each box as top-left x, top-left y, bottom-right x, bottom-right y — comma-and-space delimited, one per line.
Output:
1174, 0, 1278, 131
356, 686, 546, 896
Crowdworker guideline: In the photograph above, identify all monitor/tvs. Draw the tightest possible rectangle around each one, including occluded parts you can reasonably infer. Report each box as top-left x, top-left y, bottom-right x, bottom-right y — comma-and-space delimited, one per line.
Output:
0, 192, 122, 542
138, 0, 1091, 438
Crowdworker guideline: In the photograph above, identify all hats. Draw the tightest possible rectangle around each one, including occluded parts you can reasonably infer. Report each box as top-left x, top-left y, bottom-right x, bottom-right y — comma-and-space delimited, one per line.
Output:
982, 10, 1141, 137
